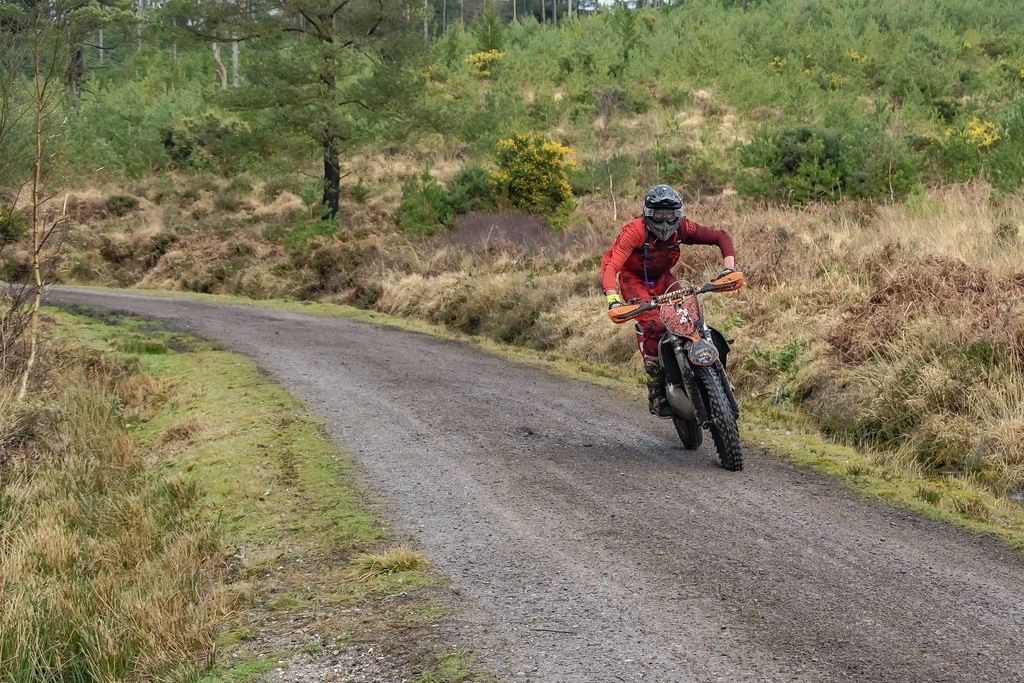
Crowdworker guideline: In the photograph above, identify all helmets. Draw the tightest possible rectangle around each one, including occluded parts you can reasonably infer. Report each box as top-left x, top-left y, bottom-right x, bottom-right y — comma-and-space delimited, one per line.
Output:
643, 184, 682, 241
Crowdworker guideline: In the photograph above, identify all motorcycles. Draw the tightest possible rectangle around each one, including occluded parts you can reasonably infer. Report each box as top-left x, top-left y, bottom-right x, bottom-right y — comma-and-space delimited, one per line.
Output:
608, 266, 748, 472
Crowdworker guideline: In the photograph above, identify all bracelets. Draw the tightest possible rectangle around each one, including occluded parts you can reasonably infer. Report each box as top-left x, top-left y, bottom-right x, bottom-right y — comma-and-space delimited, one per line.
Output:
607, 294, 620, 304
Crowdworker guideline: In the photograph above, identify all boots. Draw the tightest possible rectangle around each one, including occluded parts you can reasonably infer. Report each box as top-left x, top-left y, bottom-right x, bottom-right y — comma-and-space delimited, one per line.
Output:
645, 365, 676, 419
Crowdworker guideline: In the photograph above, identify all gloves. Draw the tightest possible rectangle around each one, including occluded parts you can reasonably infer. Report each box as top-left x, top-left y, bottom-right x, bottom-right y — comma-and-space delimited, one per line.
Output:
609, 301, 623, 310
711, 269, 736, 288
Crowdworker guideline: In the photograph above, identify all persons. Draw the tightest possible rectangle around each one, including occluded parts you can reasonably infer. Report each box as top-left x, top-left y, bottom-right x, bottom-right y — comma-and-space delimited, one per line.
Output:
602, 184, 736, 419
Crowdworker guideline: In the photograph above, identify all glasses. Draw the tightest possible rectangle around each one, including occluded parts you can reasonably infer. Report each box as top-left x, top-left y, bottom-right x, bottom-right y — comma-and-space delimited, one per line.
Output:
647, 208, 678, 224
